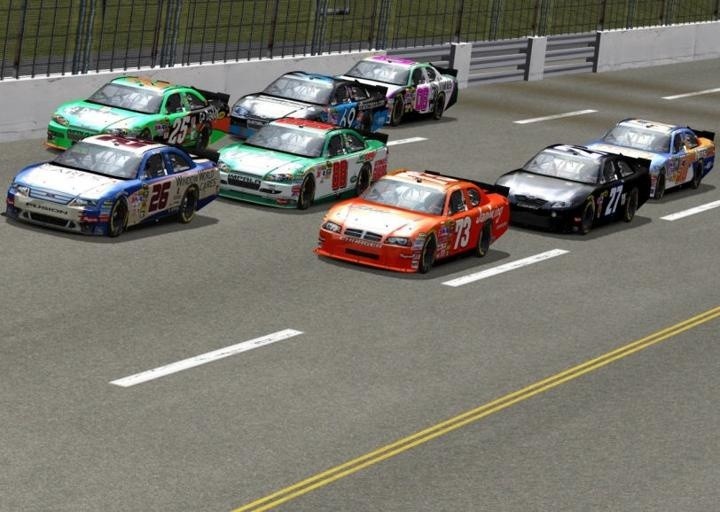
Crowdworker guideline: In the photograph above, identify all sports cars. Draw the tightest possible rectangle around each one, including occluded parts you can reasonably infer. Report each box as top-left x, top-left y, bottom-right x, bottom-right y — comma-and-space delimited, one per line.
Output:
494, 116, 716, 235
311, 166, 512, 275
